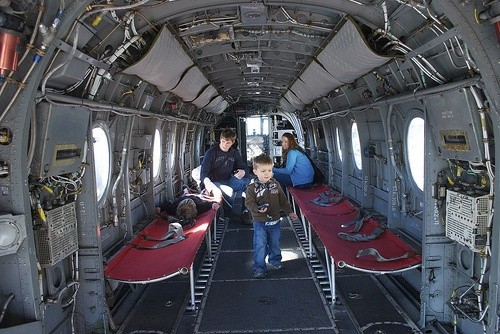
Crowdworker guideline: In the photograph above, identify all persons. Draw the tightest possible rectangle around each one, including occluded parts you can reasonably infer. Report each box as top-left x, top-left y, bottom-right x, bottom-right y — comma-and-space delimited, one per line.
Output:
243, 153, 298, 279
201, 128, 253, 224
155, 184, 217, 221
273, 133, 315, 215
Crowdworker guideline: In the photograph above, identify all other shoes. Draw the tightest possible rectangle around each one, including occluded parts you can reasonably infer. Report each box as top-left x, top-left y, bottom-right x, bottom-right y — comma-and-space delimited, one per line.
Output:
254, 270, 265, 279
272, 263, 281, 269
241, 215, 253, 224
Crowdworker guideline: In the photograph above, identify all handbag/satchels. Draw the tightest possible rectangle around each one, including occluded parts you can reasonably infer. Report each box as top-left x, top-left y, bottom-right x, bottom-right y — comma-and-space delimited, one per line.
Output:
313, 165, 324, 184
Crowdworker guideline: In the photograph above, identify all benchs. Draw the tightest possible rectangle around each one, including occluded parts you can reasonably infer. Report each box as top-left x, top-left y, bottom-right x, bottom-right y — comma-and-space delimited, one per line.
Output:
285, 183, 422, 304
103, 192, 223, 312
